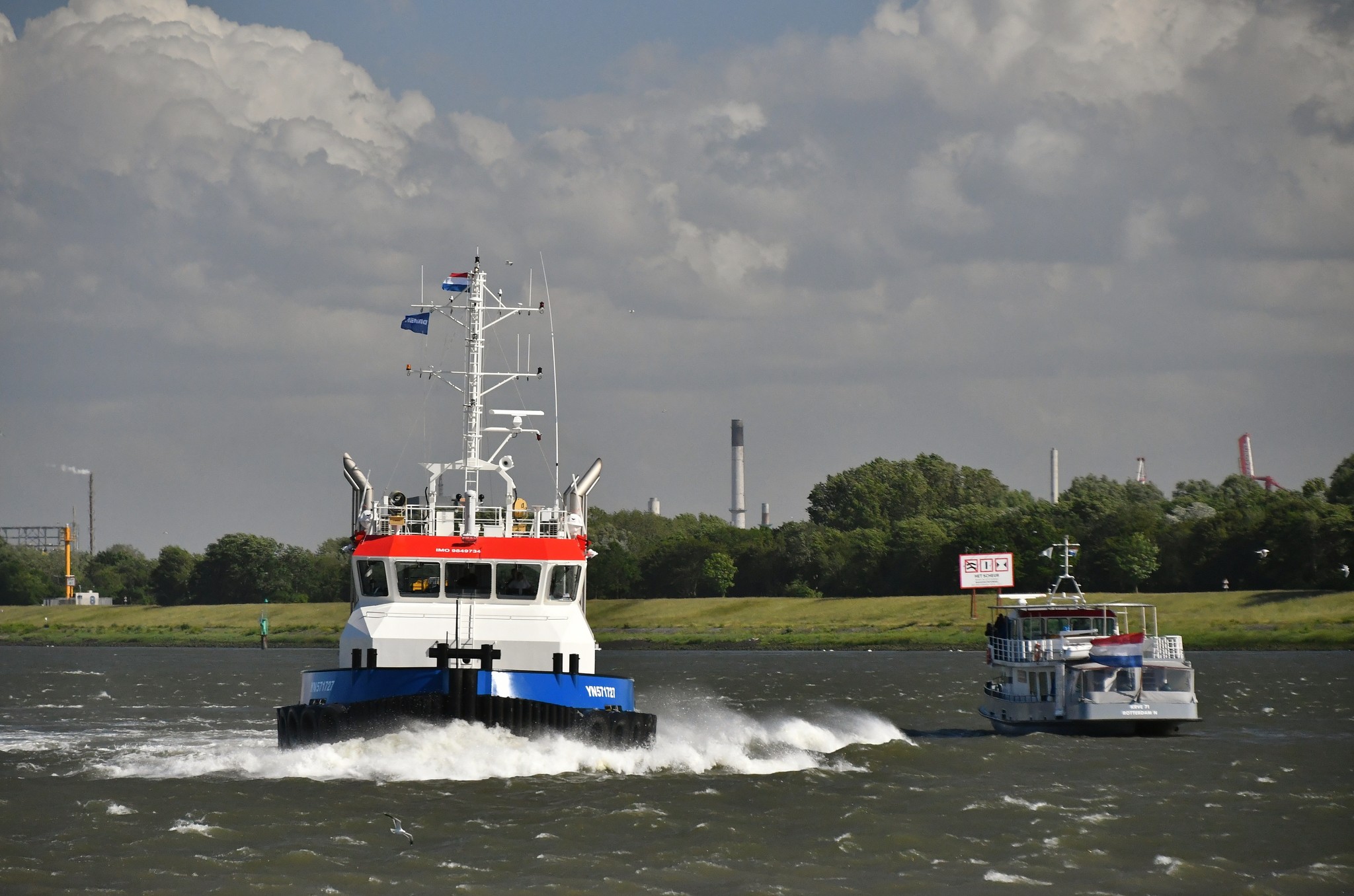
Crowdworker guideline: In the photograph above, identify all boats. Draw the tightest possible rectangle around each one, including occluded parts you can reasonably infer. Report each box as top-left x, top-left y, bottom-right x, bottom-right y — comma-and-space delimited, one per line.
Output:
978, 534, 1203, 735
275, 249, 659, 750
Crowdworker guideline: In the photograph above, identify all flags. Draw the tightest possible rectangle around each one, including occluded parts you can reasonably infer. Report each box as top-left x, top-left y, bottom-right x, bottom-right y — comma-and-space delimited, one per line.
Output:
1089, 632, 1145, 668
1038, 547, 1054, 559
442, 272, 471, 293
1068, 547, 1079, 556
401, 312, 430, 335
